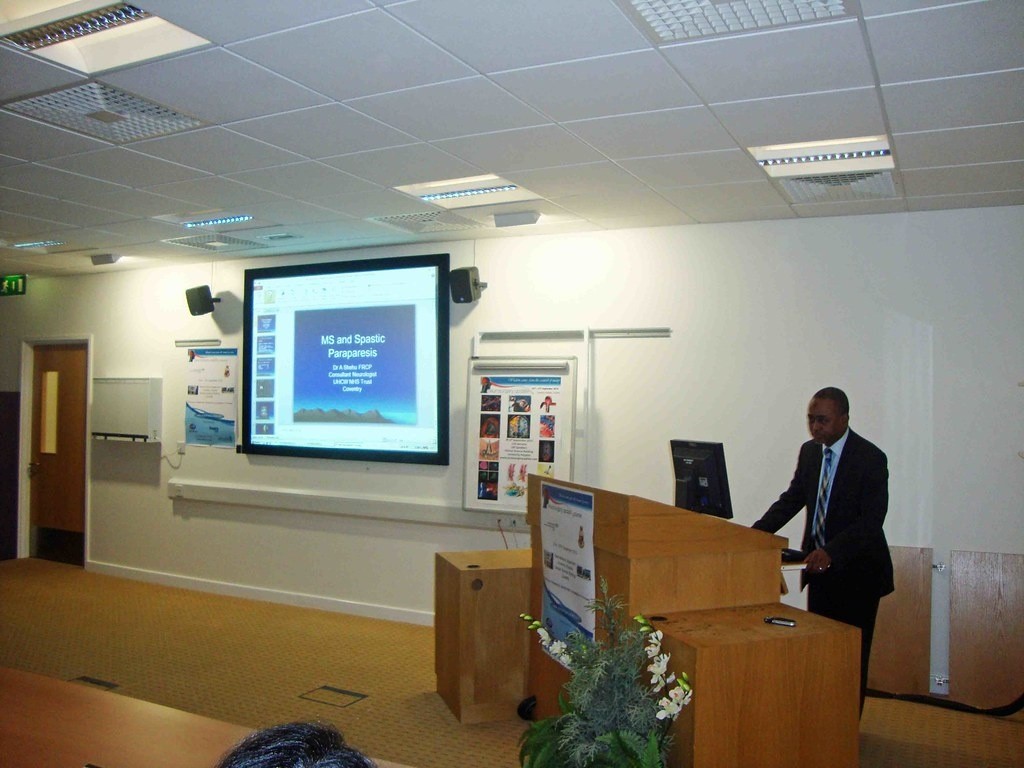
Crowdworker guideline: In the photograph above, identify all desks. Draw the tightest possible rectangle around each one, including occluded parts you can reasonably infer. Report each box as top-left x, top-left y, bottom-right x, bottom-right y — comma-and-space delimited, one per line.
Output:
0, 666, 414, 768
435, 549, 532, 726
631, 602, 861, 768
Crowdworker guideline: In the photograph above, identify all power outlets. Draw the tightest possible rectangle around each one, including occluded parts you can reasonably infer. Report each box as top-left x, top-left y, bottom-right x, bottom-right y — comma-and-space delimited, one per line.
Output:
177, 441, 185, 454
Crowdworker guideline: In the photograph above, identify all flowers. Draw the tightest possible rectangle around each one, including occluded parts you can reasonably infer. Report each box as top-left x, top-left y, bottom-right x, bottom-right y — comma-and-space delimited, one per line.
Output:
520, 575, 692, 768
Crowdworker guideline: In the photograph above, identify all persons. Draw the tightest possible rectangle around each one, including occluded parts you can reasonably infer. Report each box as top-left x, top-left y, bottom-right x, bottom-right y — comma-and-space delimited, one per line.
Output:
751, 387, 896, 723
214, 720, 378, 768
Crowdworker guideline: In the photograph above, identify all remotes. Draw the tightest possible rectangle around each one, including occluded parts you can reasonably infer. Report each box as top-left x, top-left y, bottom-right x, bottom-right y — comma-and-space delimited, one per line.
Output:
765, 615, 798, 627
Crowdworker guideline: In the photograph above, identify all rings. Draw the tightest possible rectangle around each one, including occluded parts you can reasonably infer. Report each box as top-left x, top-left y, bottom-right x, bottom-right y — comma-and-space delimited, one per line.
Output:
818, 566, 823, 571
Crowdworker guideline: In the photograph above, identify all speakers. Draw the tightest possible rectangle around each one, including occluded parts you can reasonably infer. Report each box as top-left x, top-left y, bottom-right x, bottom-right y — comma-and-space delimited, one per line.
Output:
186, 285, 214, 317
450, 266, 482, 303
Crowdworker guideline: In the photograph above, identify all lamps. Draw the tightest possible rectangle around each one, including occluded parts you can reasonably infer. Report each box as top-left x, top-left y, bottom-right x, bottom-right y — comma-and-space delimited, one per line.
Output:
494, 210, 541, 227
91, 254, 121, 265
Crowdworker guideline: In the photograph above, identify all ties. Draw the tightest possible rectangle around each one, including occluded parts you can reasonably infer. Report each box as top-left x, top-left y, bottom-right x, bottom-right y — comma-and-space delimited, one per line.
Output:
815, 448, 832, 550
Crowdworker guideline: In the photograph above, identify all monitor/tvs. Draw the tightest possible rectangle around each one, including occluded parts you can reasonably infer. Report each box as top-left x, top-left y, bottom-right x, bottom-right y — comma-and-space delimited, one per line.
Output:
670, 439, 733, 520
